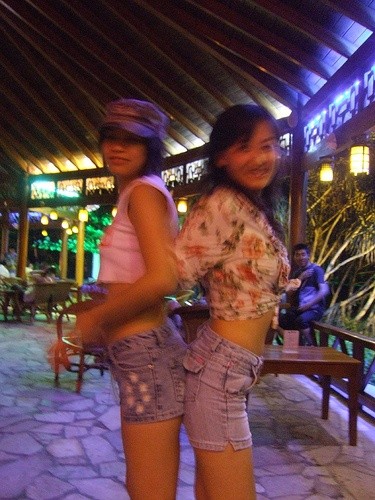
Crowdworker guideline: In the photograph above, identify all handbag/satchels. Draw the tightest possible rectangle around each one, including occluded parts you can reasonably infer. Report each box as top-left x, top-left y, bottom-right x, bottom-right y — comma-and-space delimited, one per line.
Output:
311, 263, 334, 310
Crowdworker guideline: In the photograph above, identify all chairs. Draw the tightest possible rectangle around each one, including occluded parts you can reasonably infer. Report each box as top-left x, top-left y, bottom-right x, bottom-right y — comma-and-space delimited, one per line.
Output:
55, 299, 109, 394
173, 304, 273, 346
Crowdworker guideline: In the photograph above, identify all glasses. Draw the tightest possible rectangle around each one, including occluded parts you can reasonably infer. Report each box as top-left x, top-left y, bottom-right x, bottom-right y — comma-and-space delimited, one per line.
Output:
294, 253, 307, 259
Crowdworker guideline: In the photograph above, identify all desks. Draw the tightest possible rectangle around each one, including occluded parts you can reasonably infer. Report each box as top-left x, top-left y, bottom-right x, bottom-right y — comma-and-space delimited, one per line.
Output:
246, 346, 362, 447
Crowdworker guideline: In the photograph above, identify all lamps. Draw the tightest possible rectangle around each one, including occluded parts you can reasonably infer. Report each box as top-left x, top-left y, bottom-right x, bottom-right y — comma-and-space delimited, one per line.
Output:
349, 143, 370, 177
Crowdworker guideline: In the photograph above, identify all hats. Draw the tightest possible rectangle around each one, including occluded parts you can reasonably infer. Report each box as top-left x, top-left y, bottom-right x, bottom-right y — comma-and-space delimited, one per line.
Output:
99, 97, 170, 140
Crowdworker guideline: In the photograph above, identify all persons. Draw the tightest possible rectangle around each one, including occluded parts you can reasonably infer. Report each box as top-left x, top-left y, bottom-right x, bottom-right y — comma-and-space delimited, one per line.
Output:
0, 247, 60, 304
67, 100, 189, 500
82, 244, 329, 355
48, 104, 303, 500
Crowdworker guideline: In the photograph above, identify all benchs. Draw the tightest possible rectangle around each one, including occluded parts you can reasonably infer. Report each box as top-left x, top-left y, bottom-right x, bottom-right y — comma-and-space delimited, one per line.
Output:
2, 282, 72, 324
